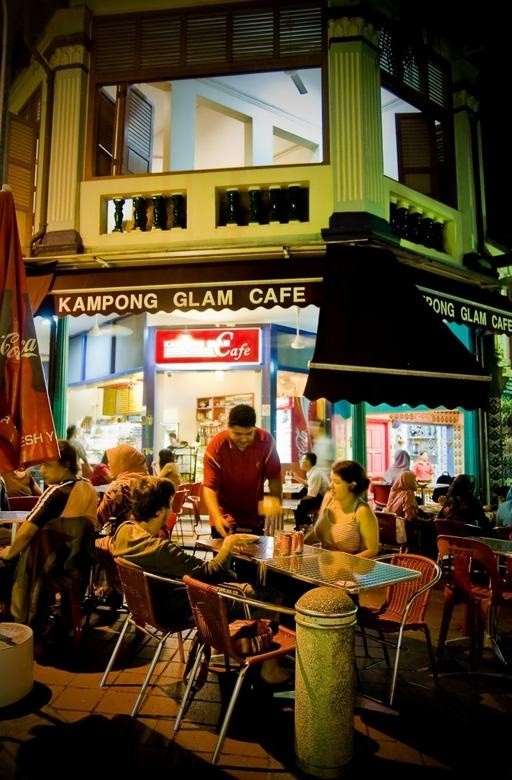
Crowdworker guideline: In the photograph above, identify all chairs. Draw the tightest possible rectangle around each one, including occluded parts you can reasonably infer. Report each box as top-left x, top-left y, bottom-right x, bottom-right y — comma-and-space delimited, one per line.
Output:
166, 573, 296, 765
160, 511, 177, 539
433, 516, 480, 544
178, 481, 203, 528
434, 533, 512, 658
172, 489, 191, 540
359, 553, 443, 706
98, 556, 197, 704
41, 574, 88, 645
23, 492, 41, 514
374, 510, 409, 554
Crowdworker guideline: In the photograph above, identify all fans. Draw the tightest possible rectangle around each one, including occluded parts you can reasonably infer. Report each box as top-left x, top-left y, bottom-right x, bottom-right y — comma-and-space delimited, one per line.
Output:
282, 70, 309, 95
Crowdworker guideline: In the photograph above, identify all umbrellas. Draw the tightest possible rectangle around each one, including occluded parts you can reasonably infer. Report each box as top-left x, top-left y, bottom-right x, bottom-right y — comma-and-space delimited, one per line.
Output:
0, 184, 61, 468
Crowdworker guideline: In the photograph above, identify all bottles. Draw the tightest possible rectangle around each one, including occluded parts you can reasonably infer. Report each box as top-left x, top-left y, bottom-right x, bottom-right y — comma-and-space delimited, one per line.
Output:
281, 532, 292, 556
291, 532, 304, 553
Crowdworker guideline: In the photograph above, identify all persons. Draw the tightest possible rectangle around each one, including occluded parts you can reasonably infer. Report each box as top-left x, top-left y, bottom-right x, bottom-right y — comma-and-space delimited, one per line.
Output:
291, 453, 328, 532
304, 460, 380, 558
151, 448, 182, 489
109, 477, 255, 629
386, 471, 419, 544
413, 451, 435, 485
385, 451, 410, 482
167, 433, 183, 457
93, 450, 113, 485
204, 403, 285, 557
438, 475, 492, 529
0, 441, 99, 621
1, 469, 41, 497
66, 426, 93, 480
86, 444, 150, 606
